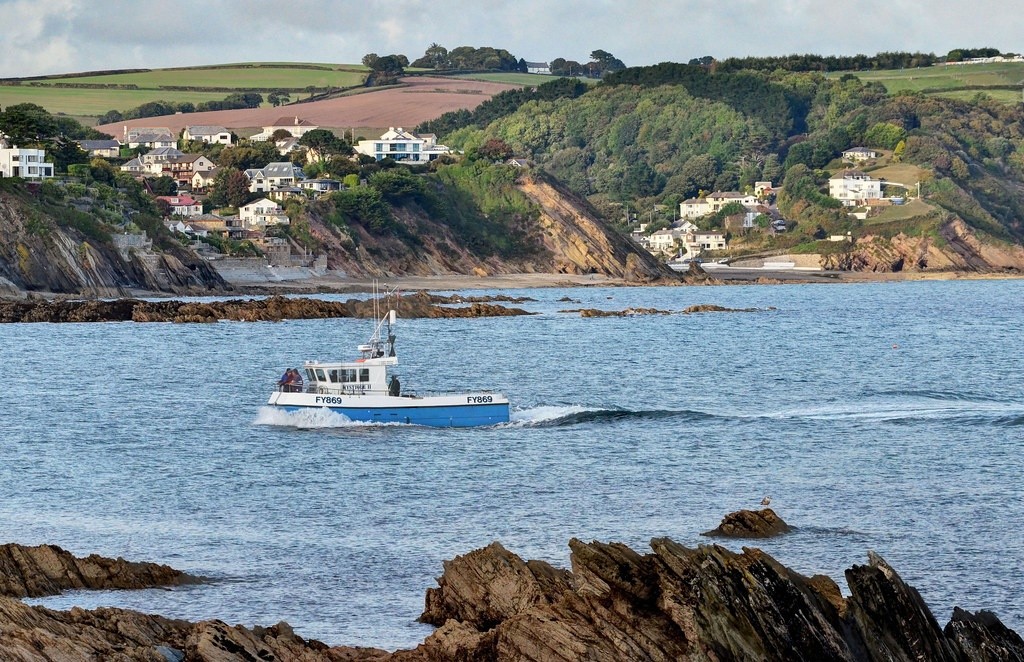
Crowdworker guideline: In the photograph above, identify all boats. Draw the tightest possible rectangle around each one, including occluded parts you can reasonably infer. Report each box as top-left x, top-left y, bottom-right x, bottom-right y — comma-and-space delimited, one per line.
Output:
265, 277, 510, 428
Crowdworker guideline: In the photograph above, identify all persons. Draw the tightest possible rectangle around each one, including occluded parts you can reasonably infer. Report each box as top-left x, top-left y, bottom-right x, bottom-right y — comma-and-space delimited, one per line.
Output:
282, 368, 292, 393
278, 368, 303, 392
331, 371, 341, 382
388, 375, 400, 396
